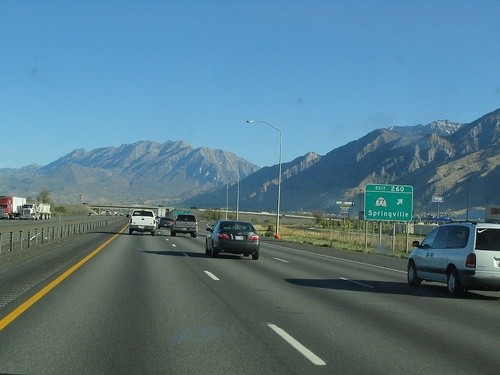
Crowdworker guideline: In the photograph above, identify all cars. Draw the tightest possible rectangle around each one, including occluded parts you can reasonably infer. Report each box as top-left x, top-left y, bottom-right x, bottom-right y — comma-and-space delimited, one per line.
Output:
88, 212, 93, 217
158, 217, 175, 229
205, 220, 260, 260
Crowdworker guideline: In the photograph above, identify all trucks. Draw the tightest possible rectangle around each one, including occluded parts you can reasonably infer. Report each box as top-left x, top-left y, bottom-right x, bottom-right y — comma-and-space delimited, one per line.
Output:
0, 196, 27, 221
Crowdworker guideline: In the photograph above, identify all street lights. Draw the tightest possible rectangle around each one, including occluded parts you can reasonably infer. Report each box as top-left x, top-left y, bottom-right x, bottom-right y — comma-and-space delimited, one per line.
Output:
217, 161, 239, 221
245, 119, 283, 238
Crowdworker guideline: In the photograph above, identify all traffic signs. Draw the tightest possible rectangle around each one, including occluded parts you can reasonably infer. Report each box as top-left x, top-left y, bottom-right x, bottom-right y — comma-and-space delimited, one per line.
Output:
363, 184, 414, 222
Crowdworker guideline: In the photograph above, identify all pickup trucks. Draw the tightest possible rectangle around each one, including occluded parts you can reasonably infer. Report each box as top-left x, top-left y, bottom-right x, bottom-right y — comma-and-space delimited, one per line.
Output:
126, 209, 161, 236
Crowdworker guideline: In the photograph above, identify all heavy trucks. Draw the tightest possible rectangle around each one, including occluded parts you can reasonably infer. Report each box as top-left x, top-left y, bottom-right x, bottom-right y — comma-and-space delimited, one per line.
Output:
18, 203, 52, 220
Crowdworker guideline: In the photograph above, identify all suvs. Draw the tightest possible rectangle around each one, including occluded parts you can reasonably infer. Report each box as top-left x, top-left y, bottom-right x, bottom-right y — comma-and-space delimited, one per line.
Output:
406, 219, 500, 298
171, 213, 199, 238
